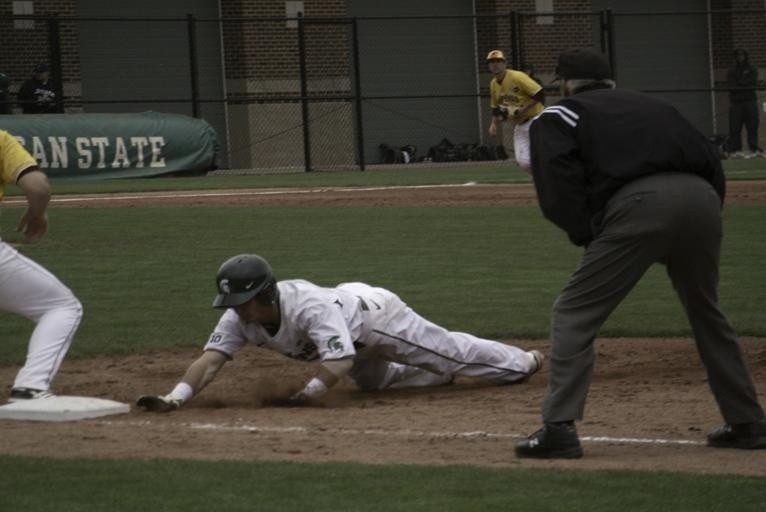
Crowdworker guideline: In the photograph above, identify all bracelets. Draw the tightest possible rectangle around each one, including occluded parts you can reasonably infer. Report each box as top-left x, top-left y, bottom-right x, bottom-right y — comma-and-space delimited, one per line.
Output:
173, 382, 193, 402
303, 378, 327, 398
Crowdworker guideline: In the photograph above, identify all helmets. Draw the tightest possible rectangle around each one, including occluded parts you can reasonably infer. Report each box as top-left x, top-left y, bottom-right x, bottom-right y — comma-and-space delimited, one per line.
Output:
211, 254, 278, 309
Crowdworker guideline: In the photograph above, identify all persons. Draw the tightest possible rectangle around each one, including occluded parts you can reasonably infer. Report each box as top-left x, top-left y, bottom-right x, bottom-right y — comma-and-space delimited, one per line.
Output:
18, 64, 64, 114
0, 127, 83, 398
485, 50, 544, 174
136, 253, 545, 415
514, 45, 766, 458
730, 50, 758, 154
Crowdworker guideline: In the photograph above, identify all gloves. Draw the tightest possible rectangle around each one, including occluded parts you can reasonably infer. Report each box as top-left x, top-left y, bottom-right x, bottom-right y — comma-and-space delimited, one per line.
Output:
261, 377, 328, 408
134, 383, 194, 411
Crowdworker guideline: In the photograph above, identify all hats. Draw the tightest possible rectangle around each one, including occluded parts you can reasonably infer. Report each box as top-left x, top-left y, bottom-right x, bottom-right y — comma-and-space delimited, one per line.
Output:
484, 49, 507, 64
553, 45, 613, 81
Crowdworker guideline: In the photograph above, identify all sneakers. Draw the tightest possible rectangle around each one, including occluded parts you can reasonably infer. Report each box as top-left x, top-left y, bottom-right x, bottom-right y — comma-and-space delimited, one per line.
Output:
515, 422, 583, 459
4, 387, 58, 403
706, 419, 766, 449
